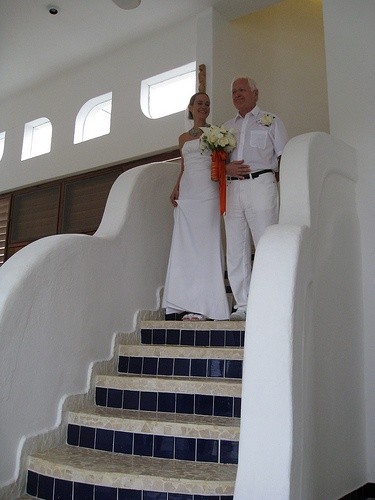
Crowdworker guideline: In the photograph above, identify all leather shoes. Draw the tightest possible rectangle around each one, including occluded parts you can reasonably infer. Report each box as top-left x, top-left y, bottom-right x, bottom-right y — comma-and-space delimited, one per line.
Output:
230, 310, 246, 321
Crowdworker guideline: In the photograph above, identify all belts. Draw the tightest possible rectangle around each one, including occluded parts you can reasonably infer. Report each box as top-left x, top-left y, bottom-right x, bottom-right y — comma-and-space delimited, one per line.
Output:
226, 169, 273, 181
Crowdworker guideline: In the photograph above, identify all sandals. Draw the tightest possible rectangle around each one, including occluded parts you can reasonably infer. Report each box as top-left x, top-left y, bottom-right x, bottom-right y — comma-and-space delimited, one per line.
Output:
181, 313, 207, 320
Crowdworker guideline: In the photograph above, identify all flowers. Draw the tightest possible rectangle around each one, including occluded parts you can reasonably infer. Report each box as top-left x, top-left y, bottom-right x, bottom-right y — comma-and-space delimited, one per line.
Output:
199, 124, 240, 217
256, 113, 277, 127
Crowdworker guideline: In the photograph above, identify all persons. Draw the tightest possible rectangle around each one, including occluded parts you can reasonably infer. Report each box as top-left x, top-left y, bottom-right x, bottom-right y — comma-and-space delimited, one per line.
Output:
218, 74, 289, 320
162, 92, 231, 320
